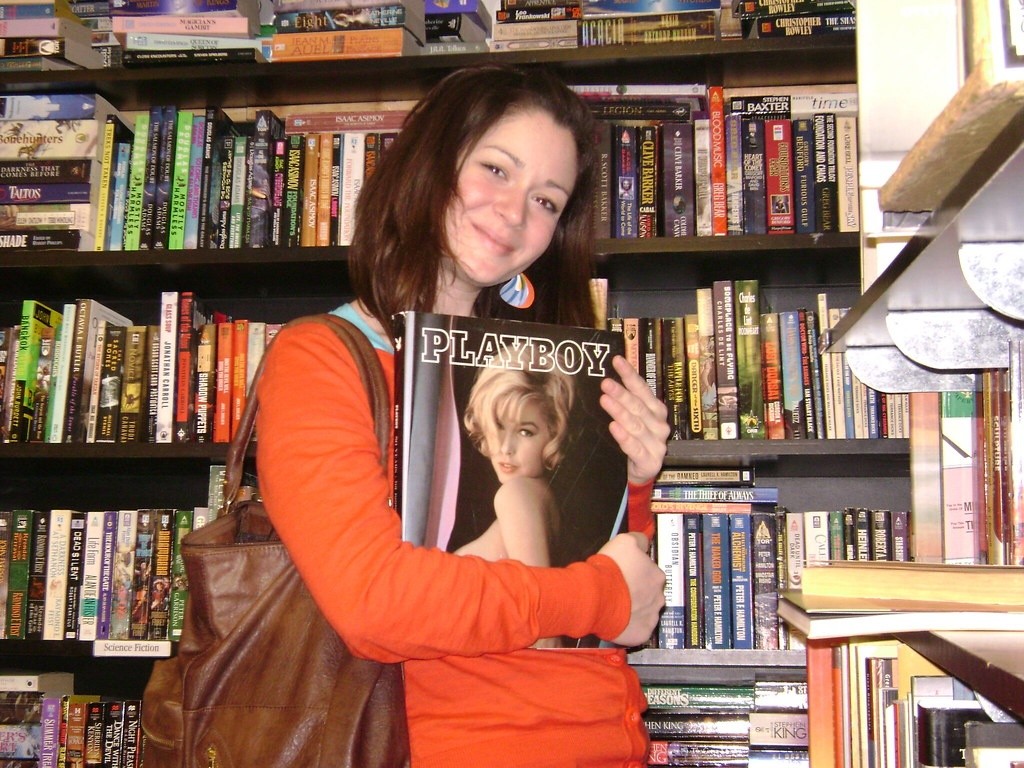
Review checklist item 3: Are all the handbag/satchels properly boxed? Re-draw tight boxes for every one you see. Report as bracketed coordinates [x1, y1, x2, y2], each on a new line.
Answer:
[142, 313, 410, 768]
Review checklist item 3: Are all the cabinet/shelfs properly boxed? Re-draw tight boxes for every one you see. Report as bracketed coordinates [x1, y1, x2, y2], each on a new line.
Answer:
[820, 61, 1024, 719]
[0, 33, 910, 768]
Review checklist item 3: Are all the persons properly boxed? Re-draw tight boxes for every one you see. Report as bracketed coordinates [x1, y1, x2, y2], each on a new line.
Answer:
[255, 64, 672, 768]
[132, 559, 151, 613]
[452, 342, 587, 647]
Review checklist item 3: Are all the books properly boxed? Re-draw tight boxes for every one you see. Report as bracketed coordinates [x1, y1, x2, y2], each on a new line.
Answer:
[2, 0, 1024, 766]
[387, 309, 628, 649]
